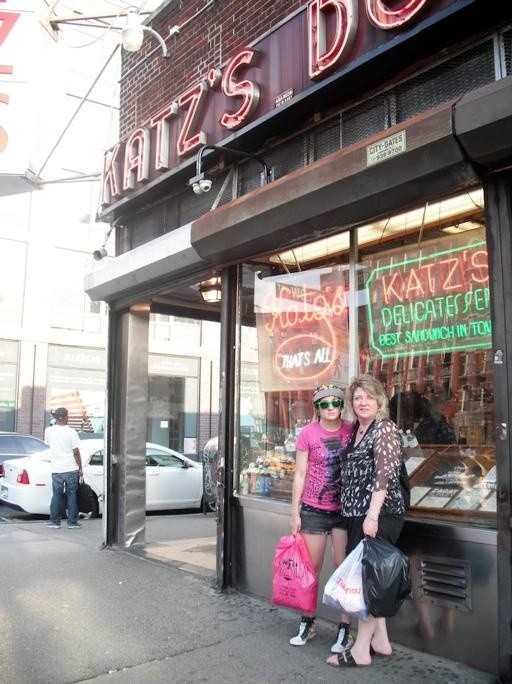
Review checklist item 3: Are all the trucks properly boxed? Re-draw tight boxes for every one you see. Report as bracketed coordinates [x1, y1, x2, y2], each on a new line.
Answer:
[240, 414, 260, 457]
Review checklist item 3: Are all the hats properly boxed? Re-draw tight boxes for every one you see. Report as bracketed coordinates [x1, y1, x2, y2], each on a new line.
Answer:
[311, 384, 345, 404]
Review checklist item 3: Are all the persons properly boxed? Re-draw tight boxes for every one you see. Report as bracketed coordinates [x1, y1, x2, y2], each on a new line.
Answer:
[327, 375, 407, 668]
[43, 406, 85, 529]
[285, 385, 359, 652]
[391, 389, 457, 635]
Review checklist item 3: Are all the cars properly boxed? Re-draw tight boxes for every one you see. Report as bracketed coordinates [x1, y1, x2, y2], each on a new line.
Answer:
[0, 430, 49, 468]
[1, 439, 216, 523]
[258, 440, 295, 480]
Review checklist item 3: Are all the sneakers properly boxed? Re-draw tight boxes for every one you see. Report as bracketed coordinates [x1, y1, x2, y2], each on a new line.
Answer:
[46, 522, 61, 529]
[67, 523, 83, 529]
[330, 622, 354, 653]
[289, 615, 317, 646]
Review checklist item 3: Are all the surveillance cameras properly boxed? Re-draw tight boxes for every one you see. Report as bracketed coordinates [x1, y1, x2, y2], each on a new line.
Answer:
[199, 179, 212, 192]
[192, 183, 202, 194]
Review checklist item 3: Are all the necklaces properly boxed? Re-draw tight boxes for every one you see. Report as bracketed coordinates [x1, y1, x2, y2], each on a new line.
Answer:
[358, 425, 368, 433]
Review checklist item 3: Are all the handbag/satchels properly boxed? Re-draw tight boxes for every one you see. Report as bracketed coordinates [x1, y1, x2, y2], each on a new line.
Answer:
[399, 462, 411, 509]
[62, 475, 99, 520]
[270, 534, 319, 613]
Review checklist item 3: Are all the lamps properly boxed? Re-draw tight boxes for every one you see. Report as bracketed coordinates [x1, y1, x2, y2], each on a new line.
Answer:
[94, 211, 125, 261]
[197, 268, 223, 304]
[119, 9, 172, 58]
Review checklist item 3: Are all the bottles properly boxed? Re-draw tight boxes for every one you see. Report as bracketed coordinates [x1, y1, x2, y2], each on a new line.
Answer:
[295, 419, 303, 435]
[260, 469, 272, 497]
[285, 433, 295, 457]
[397, 428, 422, 456]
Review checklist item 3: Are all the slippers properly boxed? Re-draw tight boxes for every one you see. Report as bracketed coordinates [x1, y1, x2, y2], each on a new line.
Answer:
[326, 650, 372, 667]
[370, 644, 392, 656]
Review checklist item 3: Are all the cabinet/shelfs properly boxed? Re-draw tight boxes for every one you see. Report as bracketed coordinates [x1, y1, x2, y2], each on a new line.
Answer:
[361, 333, 494, 444]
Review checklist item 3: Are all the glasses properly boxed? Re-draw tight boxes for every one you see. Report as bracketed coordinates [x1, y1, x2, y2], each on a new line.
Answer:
[316, 399, 343, 409]
